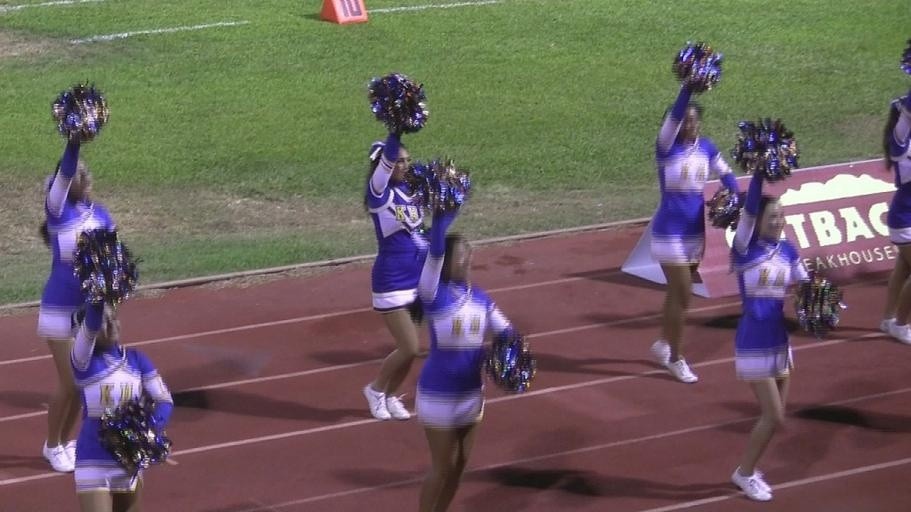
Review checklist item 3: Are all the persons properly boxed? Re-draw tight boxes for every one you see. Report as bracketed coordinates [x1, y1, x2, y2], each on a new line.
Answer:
[880, 95, 911, 344]
[69, 300, 174, 512]
[363, 131, 431, 421]
[35, 137, 117, 472]
[414, 211, 513, 512]
[731, 176, 807, 502]
[648, 84, 736, 383]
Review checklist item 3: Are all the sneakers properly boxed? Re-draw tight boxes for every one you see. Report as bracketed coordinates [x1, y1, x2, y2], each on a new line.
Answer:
[652, 340, 698, 384]
[364, 384, 410, 421]
[880, 318, 910, 344]
[731, 467, 772, 502]
[42, 440, 76, 474]
